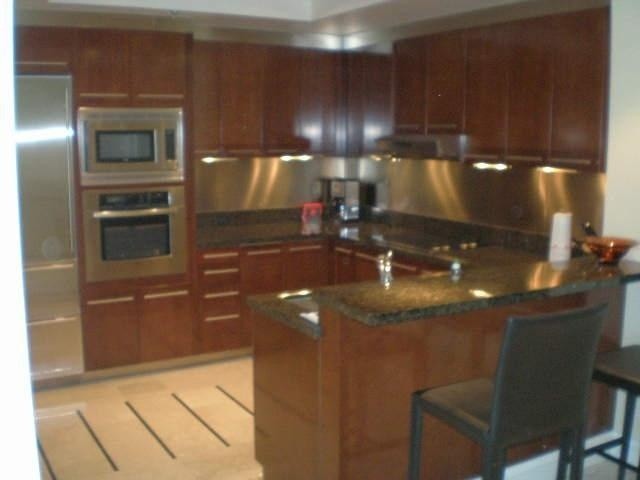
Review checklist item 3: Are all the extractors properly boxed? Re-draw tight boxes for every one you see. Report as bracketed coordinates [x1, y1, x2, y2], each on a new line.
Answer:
[374, 133, 465, 161]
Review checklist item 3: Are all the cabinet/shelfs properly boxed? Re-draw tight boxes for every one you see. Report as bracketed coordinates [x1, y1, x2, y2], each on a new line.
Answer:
[16, 26, 75, 76]
[69, 30, 192, 104]
[333, 245, 421, 291]
[185, 40, 394, 154]
[77, 275, 197, 370]
[196, 240, 331, 365]
[388, 8, 607, 170]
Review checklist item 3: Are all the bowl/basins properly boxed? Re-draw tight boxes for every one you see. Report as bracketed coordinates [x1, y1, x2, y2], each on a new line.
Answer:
[585, 236, 638, 264]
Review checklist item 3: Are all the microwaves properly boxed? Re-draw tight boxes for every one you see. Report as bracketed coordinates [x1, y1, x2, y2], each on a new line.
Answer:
[85, 118, 179, 174]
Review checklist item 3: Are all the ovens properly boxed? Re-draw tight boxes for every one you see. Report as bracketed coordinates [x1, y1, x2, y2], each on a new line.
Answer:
[80, 185, 189, 283]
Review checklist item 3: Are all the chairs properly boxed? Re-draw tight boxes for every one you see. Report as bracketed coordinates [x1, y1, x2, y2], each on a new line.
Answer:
[555, 342, 638, 480]
[410, 299, 610, 480]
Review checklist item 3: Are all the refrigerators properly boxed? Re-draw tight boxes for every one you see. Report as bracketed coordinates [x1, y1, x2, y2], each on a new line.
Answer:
[13, 73, 89, 384]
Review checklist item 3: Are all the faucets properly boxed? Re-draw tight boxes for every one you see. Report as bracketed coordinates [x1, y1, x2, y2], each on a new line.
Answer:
[373, 247, 396, 279]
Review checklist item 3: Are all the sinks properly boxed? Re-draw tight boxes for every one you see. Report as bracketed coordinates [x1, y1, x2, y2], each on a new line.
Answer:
[299, 308, 320, 325]
